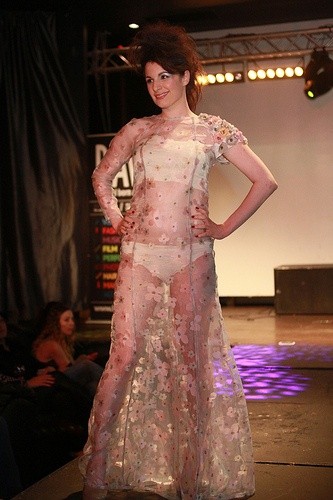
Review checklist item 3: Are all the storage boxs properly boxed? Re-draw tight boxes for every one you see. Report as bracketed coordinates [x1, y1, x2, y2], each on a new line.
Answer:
[274, 263, 333, 314]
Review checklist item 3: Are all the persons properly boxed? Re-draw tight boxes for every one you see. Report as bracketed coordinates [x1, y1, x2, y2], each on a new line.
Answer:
[24, 303, 104, 400]
[78, 22, 280, 499]
[0, 313, 89, 500]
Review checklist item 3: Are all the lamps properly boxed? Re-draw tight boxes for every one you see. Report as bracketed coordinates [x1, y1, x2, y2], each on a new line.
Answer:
[246, 56, 305, 81]
[303, 47, 333, 101]
[195, 62, 245, 87]
